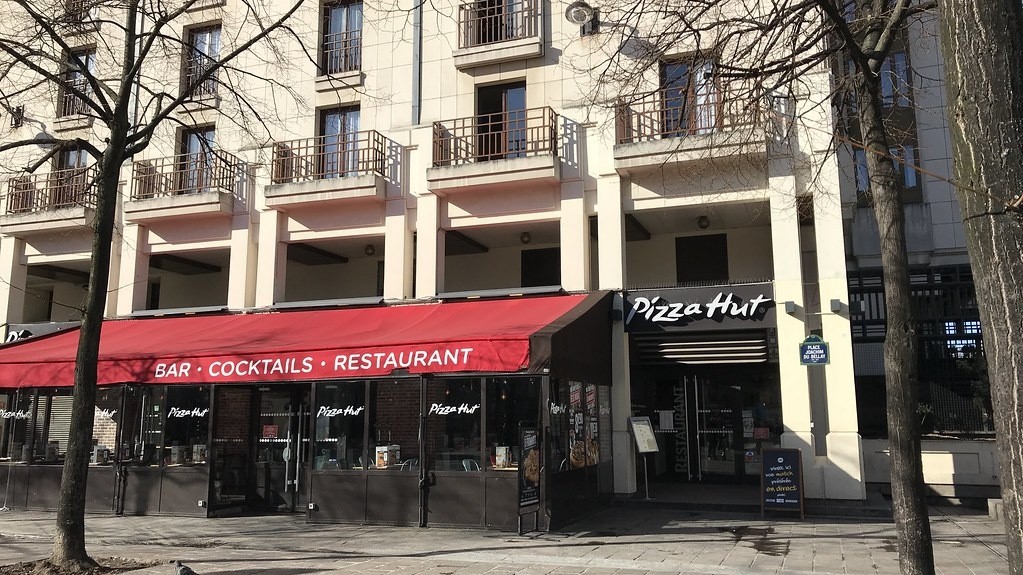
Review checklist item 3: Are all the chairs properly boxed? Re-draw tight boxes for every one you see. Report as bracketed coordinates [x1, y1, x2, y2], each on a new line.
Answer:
[461, 459, 481, 472]
[334, 452, 421, 471]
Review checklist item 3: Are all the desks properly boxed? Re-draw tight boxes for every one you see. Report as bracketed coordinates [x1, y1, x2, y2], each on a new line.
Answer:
[485, 460, 519, 471]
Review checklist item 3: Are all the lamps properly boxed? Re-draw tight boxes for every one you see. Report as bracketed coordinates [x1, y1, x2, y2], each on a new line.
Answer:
[565, 1, 601, 38]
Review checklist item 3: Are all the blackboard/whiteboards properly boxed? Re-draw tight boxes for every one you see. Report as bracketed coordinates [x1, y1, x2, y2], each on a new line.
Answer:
[759, 447, 805, 521]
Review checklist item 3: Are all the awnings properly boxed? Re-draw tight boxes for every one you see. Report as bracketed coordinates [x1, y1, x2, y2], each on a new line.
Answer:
[0, 290, 615, 389]
